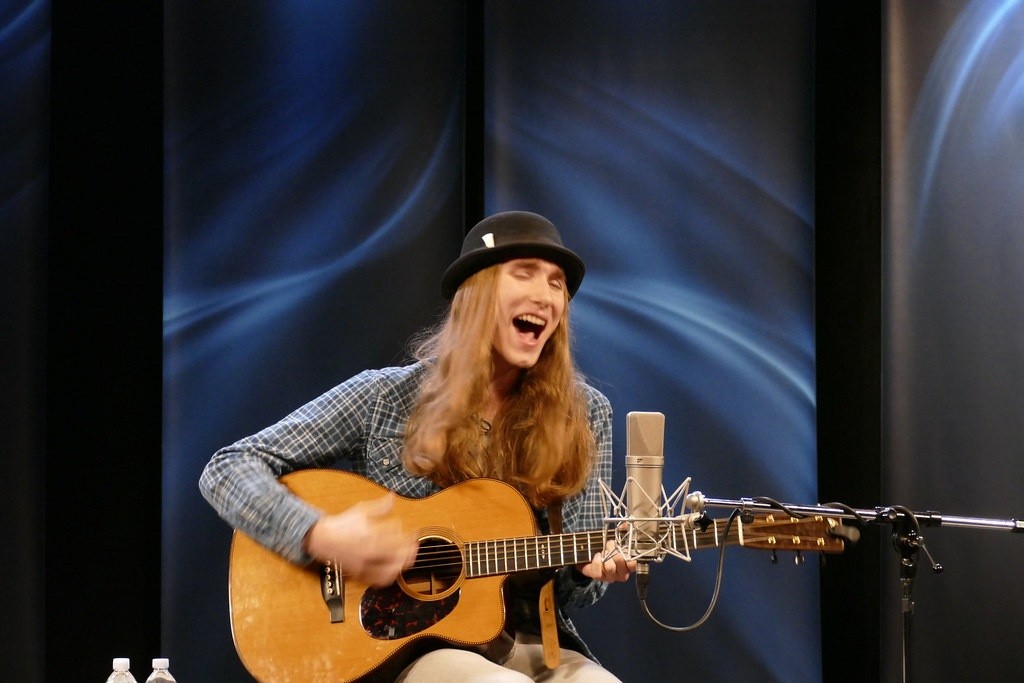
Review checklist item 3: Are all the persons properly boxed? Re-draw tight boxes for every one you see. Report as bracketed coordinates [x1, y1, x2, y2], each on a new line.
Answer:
[194, 209, 636, 682]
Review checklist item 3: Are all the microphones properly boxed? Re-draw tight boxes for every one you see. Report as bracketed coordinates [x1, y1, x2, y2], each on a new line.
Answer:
[625, 409, 665, 599]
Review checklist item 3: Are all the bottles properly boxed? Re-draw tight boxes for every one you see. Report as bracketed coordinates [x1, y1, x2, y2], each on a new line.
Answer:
[105, 657, 137, 683]
[145, 658, 177, 683]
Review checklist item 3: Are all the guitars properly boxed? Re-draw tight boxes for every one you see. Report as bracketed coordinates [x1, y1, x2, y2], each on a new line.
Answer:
[224, 466, 844, 683]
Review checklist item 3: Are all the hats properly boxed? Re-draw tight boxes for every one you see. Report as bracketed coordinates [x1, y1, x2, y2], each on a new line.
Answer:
[438, 211, 587, 302]
